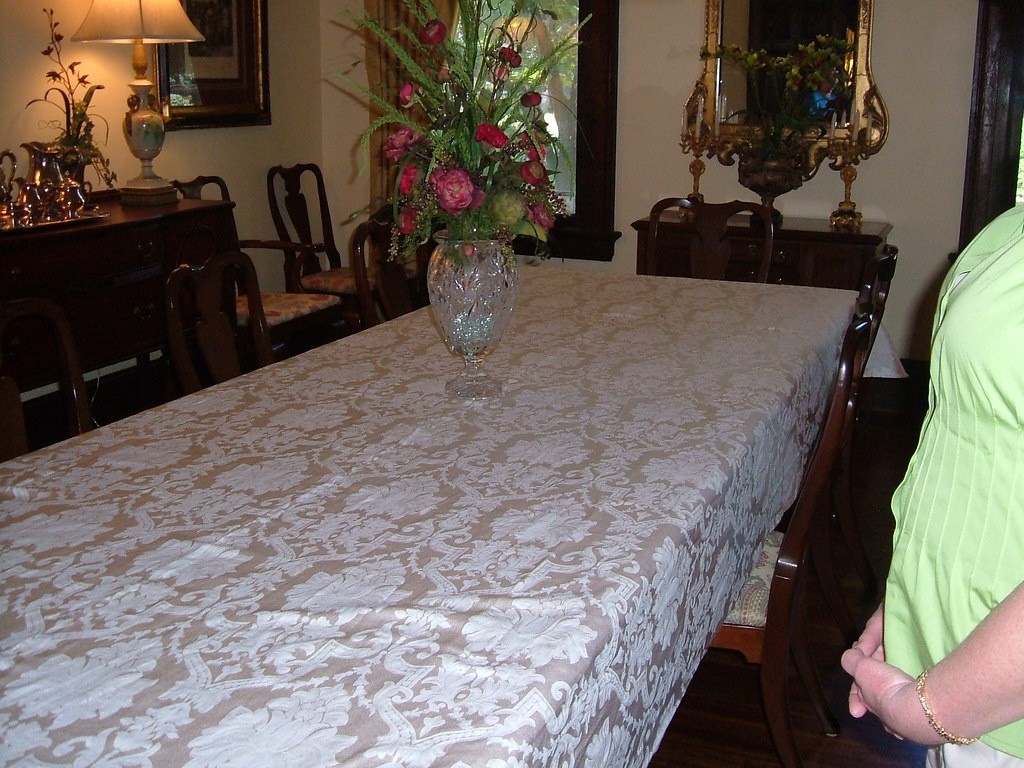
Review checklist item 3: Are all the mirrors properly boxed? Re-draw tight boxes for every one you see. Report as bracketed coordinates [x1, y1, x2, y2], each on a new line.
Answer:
[678, 0, 891, 182]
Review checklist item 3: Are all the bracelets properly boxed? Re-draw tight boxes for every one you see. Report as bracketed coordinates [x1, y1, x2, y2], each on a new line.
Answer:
[916, 670, 980, 746]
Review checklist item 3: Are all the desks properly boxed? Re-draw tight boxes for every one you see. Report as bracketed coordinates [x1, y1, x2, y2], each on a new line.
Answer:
[0, 258, 909, 768]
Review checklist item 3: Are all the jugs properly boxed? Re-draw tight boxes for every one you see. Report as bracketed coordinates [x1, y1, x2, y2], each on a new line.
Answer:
[53, 171, 85, 221]
[20, 140, 62, 223]
[12, 177, 54, 224]
[0, 147, 18, 220]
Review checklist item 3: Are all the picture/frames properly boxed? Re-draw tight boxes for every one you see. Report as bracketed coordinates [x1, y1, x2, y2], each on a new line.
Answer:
[152, 0, 272, 132]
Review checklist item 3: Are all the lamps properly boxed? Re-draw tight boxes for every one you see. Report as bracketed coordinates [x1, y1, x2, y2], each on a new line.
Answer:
[71, 0, 205, 208]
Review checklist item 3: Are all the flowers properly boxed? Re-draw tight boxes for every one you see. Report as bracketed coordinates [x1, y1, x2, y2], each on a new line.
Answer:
[23, 6, 119, 188]
[331, 0, 595, 272]
[693, 34, 859, 159]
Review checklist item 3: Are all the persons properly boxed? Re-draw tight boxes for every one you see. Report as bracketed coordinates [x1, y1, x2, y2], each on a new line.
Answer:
[841, 203, 1024, 768]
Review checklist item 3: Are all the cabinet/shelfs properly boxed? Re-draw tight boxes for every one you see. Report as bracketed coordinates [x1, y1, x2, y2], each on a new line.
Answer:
[630, 209, 893, 294]
[0, 190, 245, 395]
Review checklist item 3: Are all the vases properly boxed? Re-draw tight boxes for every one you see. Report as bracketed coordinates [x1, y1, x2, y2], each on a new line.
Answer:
[0, 142, 111, 234]
[426, 227, 517, 401]
[738, 156, 804, 230]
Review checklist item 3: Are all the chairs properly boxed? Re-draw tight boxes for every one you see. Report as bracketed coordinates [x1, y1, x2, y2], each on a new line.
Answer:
[267, 163, 419, 337]
[167, 174, 347, 368]
[349, 202, 434, 329]
[644, 197, 774, 282]
[706, 311, 873, 768]
[0, 298, 94, 464]
[806, 240, 899, 648]
